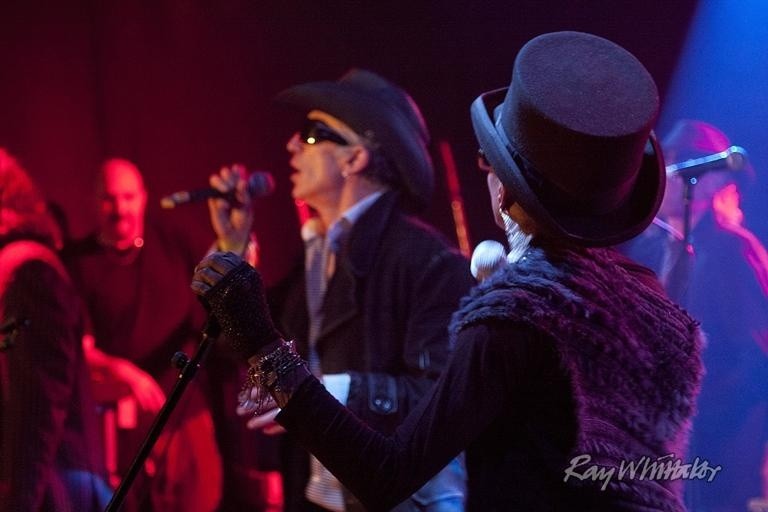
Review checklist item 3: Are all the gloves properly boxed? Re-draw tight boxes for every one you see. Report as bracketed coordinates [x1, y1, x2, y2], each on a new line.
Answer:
[188, 249, 283, 362]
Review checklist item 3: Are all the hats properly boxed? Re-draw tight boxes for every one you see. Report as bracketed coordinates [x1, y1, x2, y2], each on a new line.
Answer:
[467, 29, 670, 250]
[283, 65, 437, 199]
[661, 117, 732, 158]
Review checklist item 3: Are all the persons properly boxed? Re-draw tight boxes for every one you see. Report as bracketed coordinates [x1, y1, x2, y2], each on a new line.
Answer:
[0, 31, 768, 512]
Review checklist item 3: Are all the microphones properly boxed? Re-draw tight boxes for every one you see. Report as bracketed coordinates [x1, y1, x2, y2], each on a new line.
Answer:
[665, 146, 748, 183]
[466, 240, 507, 295]
[160, 172, 277, 209]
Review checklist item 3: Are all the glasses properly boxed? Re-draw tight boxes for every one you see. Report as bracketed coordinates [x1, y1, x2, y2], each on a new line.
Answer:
[297, 112, 352, 147]
